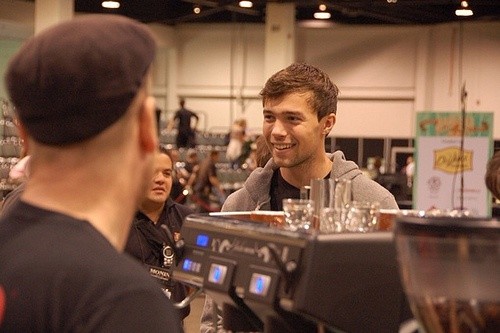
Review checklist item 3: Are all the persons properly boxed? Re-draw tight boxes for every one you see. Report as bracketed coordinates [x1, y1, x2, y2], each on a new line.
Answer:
[0, 13, 184, 333]
[200, 63, 401, 333]
[484, 149, 499, 200]
[123, 146, 194, 318]
[0, 87, 415, 212]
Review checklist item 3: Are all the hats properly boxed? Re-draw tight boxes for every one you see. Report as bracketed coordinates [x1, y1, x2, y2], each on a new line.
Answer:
[3, 13, 157, 149]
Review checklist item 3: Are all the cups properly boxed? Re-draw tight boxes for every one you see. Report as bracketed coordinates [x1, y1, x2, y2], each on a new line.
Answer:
[320, 207, 347, 234]
[283, 199, 314, 230]
[300, 178, 351, 229]
[347, 200, 380, 232]
